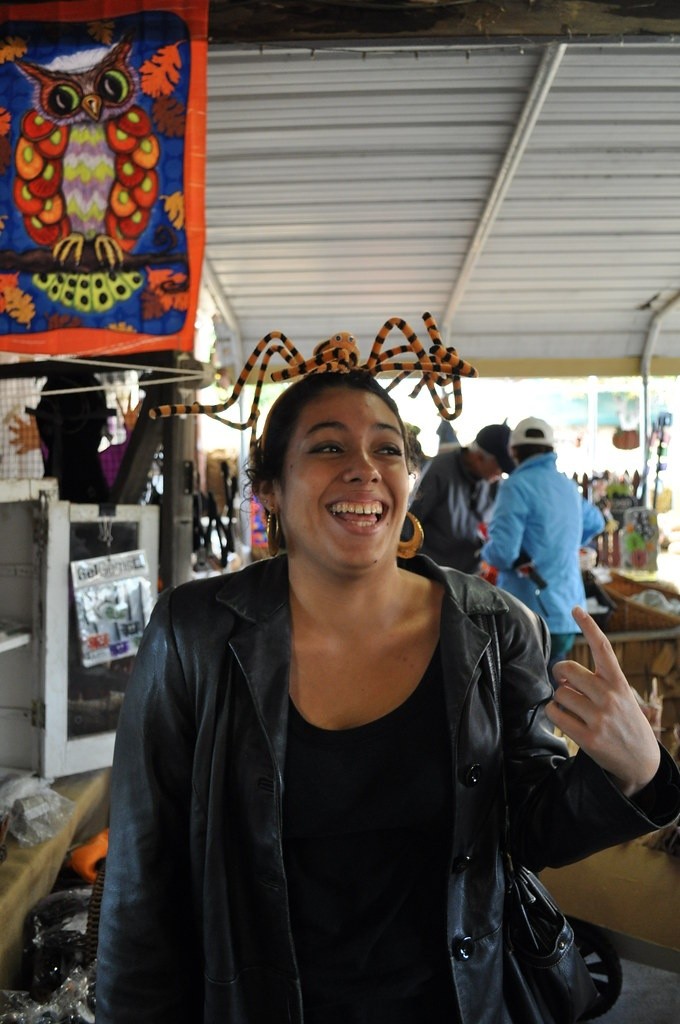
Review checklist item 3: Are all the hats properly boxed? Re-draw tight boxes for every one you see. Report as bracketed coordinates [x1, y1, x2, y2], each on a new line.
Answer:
[476, 424, 517, 474]
[507, 416, 555, 448]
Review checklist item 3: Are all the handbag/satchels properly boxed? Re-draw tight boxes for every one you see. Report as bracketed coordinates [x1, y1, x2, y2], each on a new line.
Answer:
[500, 863, 600, 1024]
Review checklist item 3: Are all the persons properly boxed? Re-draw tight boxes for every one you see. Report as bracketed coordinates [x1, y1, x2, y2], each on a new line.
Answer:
[402, 424, 518, 577]
[91, 367, 680, 1023]
[483, 416, 604, 686]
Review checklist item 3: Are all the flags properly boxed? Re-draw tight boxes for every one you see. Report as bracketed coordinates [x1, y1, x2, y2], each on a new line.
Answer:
[2, 2, 206, 355]
